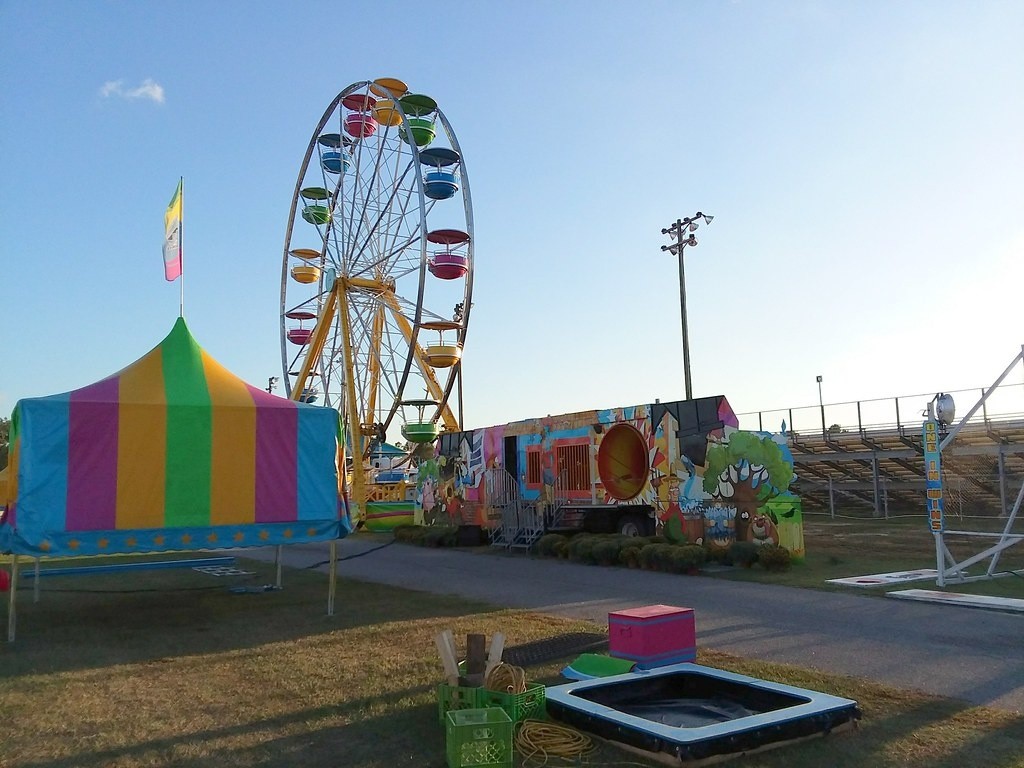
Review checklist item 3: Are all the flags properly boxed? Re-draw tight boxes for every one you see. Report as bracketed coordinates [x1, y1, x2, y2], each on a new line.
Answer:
[162, 179, 183, 281]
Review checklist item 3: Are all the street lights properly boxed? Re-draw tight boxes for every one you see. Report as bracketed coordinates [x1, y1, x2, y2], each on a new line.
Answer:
[660, 211, 715, 400]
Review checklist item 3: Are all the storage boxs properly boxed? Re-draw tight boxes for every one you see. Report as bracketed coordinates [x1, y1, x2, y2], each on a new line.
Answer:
[609, 603, 697, 670]
[438, 658, 547, 768]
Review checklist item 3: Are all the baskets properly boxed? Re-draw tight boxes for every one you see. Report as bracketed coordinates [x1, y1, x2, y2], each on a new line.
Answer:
[445, 707, 513, 768]
[475, 682, 545, 724]
[438, 676, 477, 724]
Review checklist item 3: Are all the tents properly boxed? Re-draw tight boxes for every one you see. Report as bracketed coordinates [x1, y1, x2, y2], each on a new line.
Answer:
[0, 317, 350, 641]
